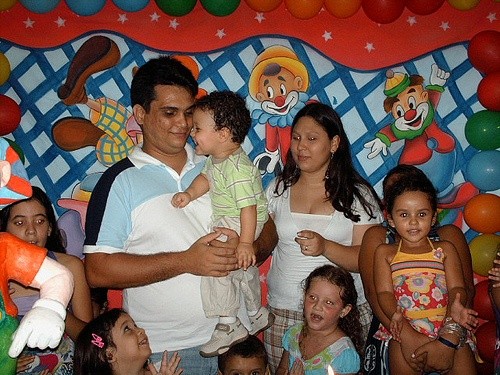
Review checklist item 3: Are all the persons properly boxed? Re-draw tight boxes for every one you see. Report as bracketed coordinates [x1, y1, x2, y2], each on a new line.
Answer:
[359, 164, 478, 375]
[263, 102, 384, 375]
[82, 56, 276, 375]
[274, 264, 365, 375]
[0, 137, 74, 375]
[218, 334, 271, 375]
[0, 183, 94, 375]
[73, 308, 183, 375]
[170, 90, 275, 358]
[488, 252, 500, 375]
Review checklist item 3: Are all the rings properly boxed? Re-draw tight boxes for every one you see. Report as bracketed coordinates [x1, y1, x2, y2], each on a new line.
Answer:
[305, 246, 308, 250]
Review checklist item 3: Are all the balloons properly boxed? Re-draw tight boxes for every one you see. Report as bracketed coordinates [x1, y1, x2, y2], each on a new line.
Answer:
[0, 0, 478, 24]
[466, 149, 500, 191]
[477, 73, 500, 110]
[467, 30, 500, 75]
[465, 110, 500, 150]
[0, 52, 25, 165]
[463, 194, 500, 234]
[468, 234, 500, 361]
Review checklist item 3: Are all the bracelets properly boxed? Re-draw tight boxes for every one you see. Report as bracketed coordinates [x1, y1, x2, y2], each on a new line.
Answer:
[438, 336, 458, 349]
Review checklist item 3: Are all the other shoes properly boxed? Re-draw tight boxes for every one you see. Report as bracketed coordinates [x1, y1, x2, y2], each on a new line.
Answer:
[199, 317, 249, 358]
[249, 306, 275, 338]
